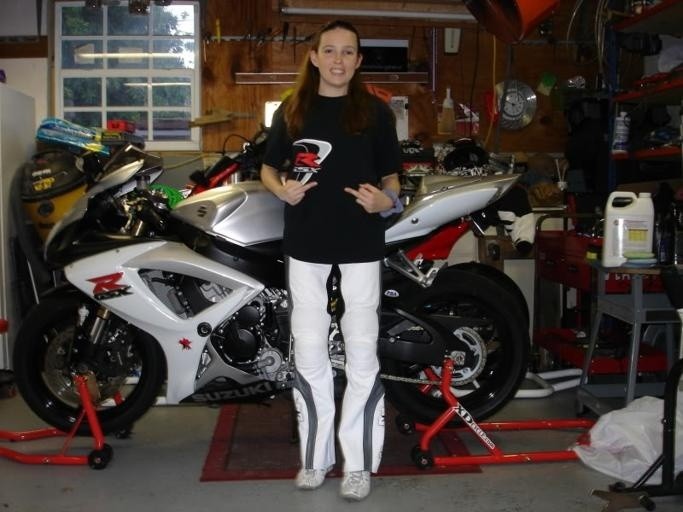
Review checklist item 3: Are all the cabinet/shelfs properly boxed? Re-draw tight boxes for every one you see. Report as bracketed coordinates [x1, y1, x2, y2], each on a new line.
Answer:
[530, 212, 664, 375]
[570, 259, 677, 420]
[605, 1, 682, 165]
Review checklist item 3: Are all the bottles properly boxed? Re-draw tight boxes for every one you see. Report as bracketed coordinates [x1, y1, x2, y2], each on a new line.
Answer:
[654, 208, 683, 266]
[440, 88, 456, 133]
[610, 112, 631, 154]
[106, 119, 135, 133]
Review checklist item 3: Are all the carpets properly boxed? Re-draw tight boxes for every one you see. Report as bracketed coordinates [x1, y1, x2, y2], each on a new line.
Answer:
[196, 396, 484, 483]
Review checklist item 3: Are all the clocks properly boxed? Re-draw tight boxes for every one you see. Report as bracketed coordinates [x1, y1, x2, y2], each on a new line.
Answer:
[485, 78, 537, 131]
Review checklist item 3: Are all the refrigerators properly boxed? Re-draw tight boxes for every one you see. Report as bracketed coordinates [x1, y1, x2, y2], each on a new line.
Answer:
[0, 80, 37, 377]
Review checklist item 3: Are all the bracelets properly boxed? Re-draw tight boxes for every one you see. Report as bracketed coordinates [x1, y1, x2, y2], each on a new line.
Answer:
[378, 185, 402, 218]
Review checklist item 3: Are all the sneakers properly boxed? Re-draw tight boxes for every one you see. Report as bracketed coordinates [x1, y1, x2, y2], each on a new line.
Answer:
[292, 461, 336, 490]
[338, 469, 373, 502]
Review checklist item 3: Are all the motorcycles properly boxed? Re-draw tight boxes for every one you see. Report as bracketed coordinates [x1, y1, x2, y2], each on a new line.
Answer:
[12, 139, 530, 433]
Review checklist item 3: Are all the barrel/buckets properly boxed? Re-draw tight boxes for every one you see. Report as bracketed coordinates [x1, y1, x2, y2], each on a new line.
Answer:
[601, 190, 654, 269]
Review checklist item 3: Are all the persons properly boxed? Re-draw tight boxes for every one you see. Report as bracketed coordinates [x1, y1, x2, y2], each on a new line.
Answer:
[257, 21, 402, 501]
